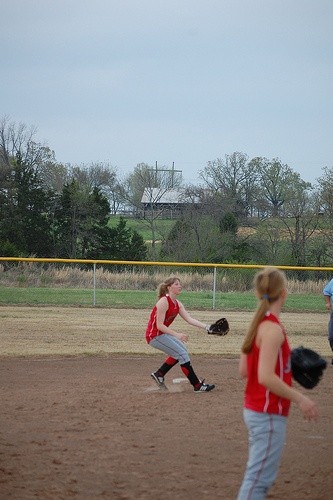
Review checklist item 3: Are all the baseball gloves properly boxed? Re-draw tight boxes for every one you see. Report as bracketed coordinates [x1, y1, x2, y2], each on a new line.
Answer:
[291, 346, 327, 390]
[208, 317, 230, 336]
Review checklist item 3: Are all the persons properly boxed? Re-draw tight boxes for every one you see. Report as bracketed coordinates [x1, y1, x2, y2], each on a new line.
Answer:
[146, 278, 215, 393]
[238, 269, 319, 500]
[323, 279, 333, 364]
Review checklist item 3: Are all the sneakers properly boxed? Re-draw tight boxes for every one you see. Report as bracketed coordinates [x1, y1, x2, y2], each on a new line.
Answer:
[194, 377, 216, 393]
[151, 368, 169, 391]
